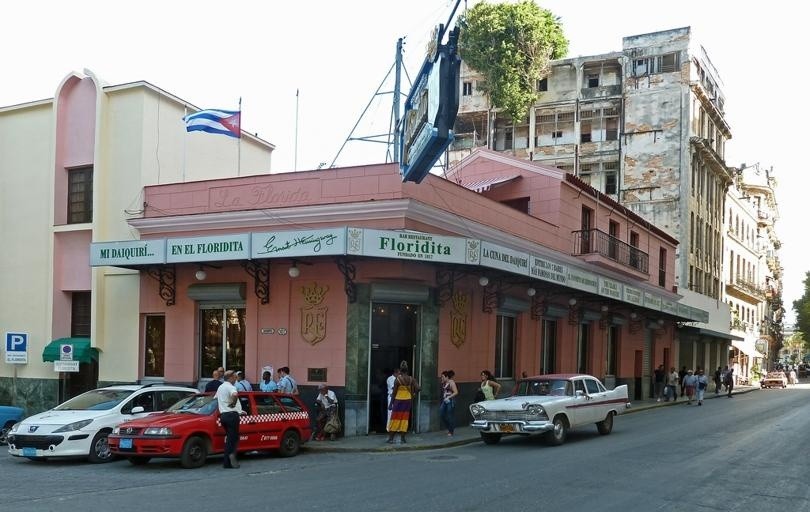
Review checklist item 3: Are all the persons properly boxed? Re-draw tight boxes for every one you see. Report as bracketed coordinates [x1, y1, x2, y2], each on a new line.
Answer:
[313, 384, 339, 441]
[385, 360, 420, 443]
[439, 369, 459, 436]
[205, 366, 300, 407]
[147, 346, 156, 374]
[385, 366, 400, 433]
[540, 384, 550, 394]
[213, 370, 243, 469]
[653, 364, 733, 406]
[788, 368, 797, 385]
[512, 371, 534, 396]
[477, 371, 501, 400]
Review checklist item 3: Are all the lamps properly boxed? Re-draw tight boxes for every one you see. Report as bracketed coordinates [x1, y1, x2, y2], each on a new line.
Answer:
[288, 259, 314, 277]
[196, 264, 222, 280]
[478, 268, 685, 339]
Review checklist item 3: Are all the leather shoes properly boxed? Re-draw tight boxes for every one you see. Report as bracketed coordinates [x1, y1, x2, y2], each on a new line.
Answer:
[230, 454, 240, 468]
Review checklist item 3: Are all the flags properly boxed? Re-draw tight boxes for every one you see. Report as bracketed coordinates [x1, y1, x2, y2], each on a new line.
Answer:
[183, 108, 240, 138]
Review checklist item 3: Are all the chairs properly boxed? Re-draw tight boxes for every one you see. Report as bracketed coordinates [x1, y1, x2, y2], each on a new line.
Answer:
[135, 394, 152, 411]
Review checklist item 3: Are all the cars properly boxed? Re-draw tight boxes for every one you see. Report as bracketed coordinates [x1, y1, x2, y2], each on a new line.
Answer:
[0, 403, 27, 447]
[105, 389, 315, 472]
[758, 363, 810, 390]
[6, 379, 203, 465]
[467, 371, 634, 448]
